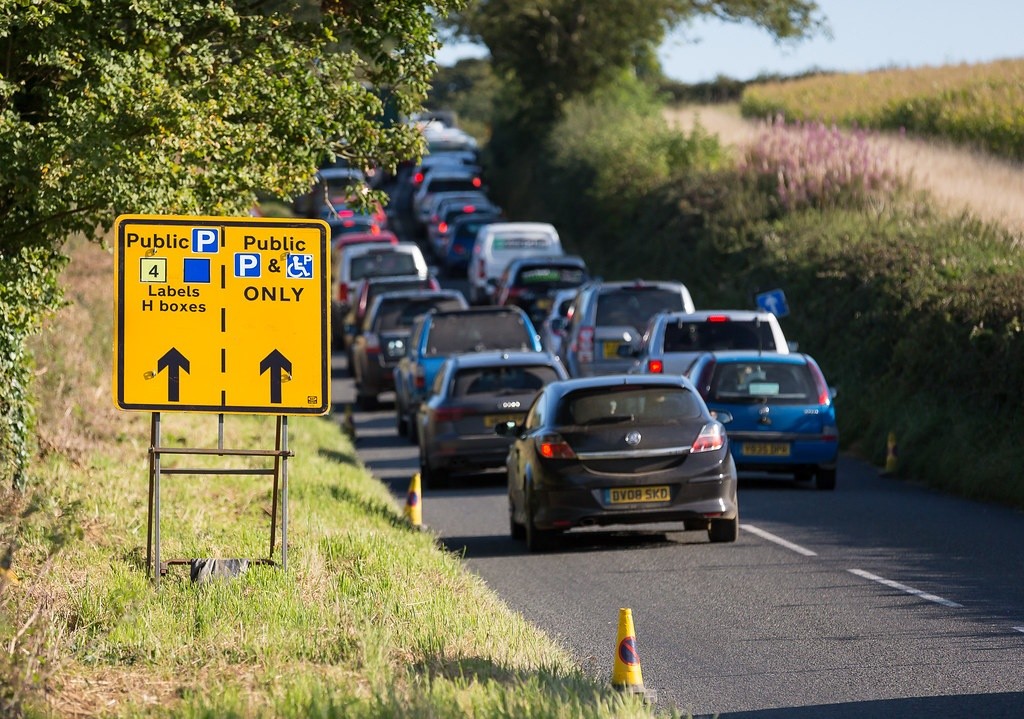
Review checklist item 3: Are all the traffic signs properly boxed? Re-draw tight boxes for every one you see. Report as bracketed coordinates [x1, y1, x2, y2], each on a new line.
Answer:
[113, 214, 331, 416]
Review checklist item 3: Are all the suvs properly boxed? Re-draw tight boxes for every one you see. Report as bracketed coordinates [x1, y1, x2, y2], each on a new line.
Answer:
[342, 290, 485, 411]
[616, 308, 800, 394]
[485, 256, 591, 335]
[494, 374, 739, 554]
[466, 221, 567, 308]
[387, 301, 541, 447]
[549, 277, 695, 383]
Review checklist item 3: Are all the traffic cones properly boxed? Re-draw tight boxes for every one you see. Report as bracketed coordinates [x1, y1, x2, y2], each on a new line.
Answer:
[879, 433, 899, 479]
[605, 606, 658, 707]
[402, 474, 427, 533]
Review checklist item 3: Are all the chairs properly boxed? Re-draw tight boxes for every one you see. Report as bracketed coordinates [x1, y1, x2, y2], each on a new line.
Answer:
[766, 367, 799, 393]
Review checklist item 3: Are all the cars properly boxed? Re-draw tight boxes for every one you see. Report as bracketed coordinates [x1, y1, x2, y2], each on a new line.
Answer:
[531, 287, 580, 369]
[411, 346, 571, 491]
[683, 348, 837, 489]
[314, 110, 504, 377]
